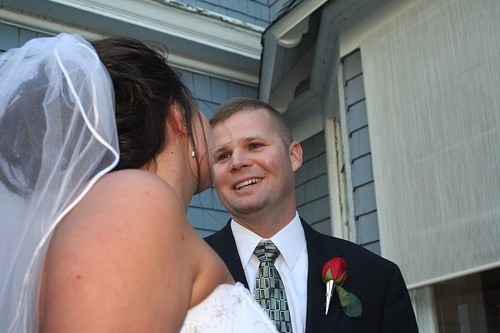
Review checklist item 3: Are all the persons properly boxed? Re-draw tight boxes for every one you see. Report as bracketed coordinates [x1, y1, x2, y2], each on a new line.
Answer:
[0, 31, 278, 333]
[203, 96, 419, 333]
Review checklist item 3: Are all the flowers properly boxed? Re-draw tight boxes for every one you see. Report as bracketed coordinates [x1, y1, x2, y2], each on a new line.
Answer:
[322, 257, 347, 285]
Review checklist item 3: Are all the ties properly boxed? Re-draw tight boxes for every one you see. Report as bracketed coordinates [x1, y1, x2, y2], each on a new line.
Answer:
[252, 239, 292, 333]
